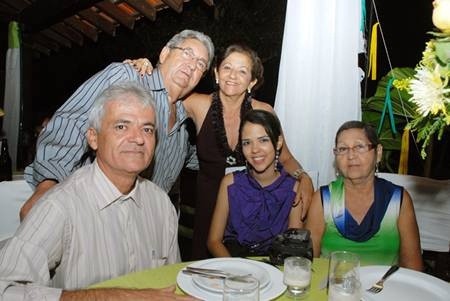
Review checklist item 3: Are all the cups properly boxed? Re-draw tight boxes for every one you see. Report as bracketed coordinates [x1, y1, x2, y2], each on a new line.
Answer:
[328, 250, 362, 301]
[283, 256, 312, 299]
[222, 275, 259, 301]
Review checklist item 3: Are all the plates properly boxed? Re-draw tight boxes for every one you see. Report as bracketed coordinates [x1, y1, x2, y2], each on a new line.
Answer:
[360, 266, 450, 301]
[192, 260, 272, 293]
[176, 257, 287, 301]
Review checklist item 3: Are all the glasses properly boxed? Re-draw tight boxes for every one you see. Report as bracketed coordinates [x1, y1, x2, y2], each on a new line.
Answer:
[331, 140, 380, 157]
[166, 44, 211, 76]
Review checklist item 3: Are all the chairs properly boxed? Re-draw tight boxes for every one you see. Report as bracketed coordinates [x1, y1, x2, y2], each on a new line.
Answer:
[0, 179, 34, 242]
[373, 173, 450, 252]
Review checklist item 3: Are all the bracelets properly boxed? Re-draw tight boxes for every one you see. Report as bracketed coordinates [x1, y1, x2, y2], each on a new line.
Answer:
[293, 169, 307, 179]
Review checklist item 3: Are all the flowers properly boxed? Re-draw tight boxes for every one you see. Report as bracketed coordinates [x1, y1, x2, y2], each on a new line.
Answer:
[407, 0, 450, 160]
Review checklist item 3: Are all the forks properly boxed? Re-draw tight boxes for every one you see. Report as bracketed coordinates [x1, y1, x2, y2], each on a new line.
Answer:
[366, 263, 400, 293]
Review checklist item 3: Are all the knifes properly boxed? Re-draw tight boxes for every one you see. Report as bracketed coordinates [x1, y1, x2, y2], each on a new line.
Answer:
[182, 270, 249, 283]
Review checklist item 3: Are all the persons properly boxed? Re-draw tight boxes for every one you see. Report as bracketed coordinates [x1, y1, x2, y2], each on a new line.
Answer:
[0, 81, 204, 301]
[305, 121, 423, 272]
[124, 46, 314, 260]
[18, 29, 215, 225]
[208, 109, 303, 258]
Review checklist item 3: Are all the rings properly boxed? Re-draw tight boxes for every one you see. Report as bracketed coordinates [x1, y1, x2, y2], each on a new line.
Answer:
[144, 62, 148, 67]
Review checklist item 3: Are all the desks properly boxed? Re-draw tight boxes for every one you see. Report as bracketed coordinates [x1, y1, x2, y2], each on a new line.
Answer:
[82, 256, 349, 301]
